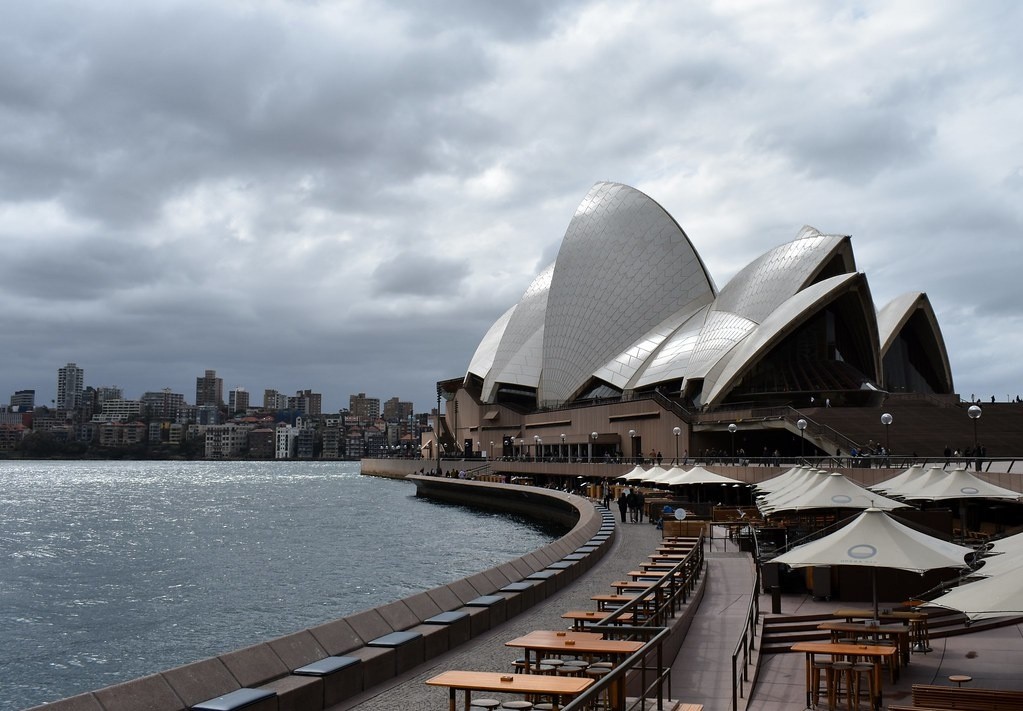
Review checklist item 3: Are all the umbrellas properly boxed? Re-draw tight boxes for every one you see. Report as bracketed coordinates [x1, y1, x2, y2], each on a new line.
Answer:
[751, 465, 1023, 645]
[617, 465, 746, 504]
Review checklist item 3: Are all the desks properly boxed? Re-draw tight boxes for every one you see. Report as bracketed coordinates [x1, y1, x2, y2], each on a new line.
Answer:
[505, 630, 646, 711]
[590, 595, 655, 619]
[639, 537, 702, 603]
[817, 622, 911, 669]
[560, 610, 634, 640]
[834, 609, 928, 650]
[627, 571, 682, 618]
[611, 580, 670, 624]
[791, 642, 897, 711]
[426, 670, 595, 711]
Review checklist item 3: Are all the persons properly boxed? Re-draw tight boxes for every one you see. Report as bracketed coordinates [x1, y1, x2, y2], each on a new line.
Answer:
[638, 449, 663, 465]
[698, 447, 782, 469]
[835, 440, 890, 468]
[517, 451, 588, 463]
[826, 397, 831, 408]
[618, 488, 645, 524]
[811, 396, 815, 408]
[944, 443, 986, 468]
[603, 487, 611, 510]
[682, 449, 687, 465]
[655, 385, 668, 398]
[414, 467, 472, 480]
[595, 395, 602, 405]
[603, 451, 623, 464]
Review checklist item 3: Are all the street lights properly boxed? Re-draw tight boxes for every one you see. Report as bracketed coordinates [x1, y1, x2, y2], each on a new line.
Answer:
[968, 405, 982, 471]
[592, 432, 598, 464]
[385, 446, 389, 458]
[629, 429, 636, 465]
[477, 441, 480, 457]
[538, 439, 541, 460]
[391, 446, 394, 459]
[797, 419, 807, 467]
[444, 443, 447, 458]
[403, 445, 407, 459]
[534, 435, 539, 462]
[510, 437, 515, 461]
[520, 440, 523, 461]
[1007, 394, 1009, 402]
[504, 441, 509, 460]
[881, 414, 893, 468]
[436, 382, 443, 473]
[410, 409, 413, 453]
[673, 427, 682, 465]
[728, 424, 737, 466]
[560, 434, 566, 464]
[465, 442, 469, 455]
[490, 441, 494, 460]
[454, 397, 459, 457]
[380, 446, 383, 459]
[411, 445, 414, 460]
[396, 445, 400, 459]
[418, 445, 422, 459]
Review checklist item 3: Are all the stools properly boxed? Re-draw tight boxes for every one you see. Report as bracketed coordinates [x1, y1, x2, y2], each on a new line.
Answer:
[470, 699, 564, 711]
[511, 658, 613, 711]
[949, 675, 972, 687]
[813, 619, 926, 711]
[903, 601, 923, 612]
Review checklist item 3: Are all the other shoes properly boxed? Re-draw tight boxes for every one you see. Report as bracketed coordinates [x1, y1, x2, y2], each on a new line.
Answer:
[630, 521, 633, 523]
[633, 518, 637, 523]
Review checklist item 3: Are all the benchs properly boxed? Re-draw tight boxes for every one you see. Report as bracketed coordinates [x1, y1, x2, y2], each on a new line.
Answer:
[888, 683, 1023, 711]
